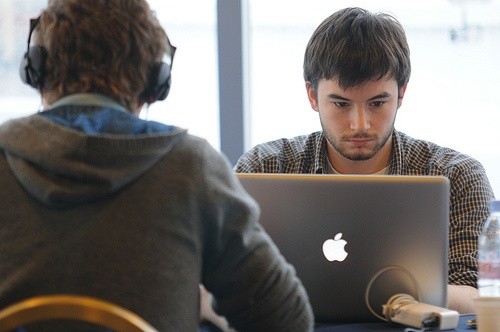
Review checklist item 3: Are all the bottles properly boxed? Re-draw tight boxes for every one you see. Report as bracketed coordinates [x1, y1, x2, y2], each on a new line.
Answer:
[476, 200, 500, 297]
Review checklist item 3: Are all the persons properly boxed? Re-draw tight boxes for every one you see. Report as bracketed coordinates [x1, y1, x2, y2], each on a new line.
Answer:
[235, 7, 495, 314]
[0, 0, 314, 332]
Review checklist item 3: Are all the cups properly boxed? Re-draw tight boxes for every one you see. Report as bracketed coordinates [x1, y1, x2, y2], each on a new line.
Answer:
[472, 298, 500, 332]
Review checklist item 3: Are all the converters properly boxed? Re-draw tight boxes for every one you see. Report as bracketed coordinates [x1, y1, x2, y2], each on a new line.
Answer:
[381, 301, 460, 331]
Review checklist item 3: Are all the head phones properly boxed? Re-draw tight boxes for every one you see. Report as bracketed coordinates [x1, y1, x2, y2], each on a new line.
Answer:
[18, 14, 176, 105]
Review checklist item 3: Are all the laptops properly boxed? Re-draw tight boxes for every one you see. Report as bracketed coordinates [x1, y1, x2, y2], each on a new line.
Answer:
[234, 172, 451, 323]
